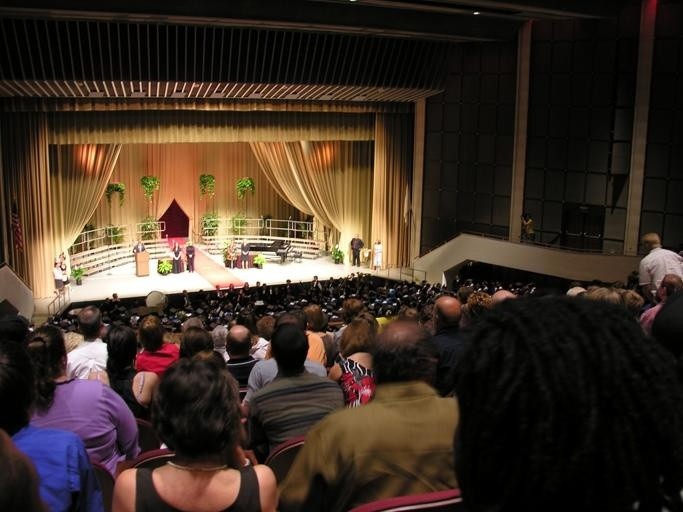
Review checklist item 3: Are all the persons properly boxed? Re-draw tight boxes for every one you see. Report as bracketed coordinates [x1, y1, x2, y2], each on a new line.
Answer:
[654, 295, 683, 354]
[226, 238, 237, 267]
[522, 214, 535, 240]
[277, 323, 460, 511]
[186, 241, 195, 272]
[0, 430, 51, 512]
[53, 260, 64, 296]
[224, 324, 258, 387]
[133, 240, 145, 252]
[372, 240, 383, 272]
[47, 273, 341, 341]
[172, 242, 182, 274]
[133, 316, 177, 378]
[66, 306, 110, 387]
[112, 355, 279, 512]
[23, 326, 142, 475]
[240, 239, 250, 269]
[639, 233, 683, 295]
[88, 328, 159, 420]
[1, 351, 106, 512]
[341, 272, 647, 327]
[329, 325, 377, 409]
[454, 296, 683, 512]
[350, 233, 364, 266]
[61, 256, 70, 286]
[178, 327, 225, 371]
[641, 274, 683, 334]
[248, 325, 344, 454]
[2, 314, 30, 352]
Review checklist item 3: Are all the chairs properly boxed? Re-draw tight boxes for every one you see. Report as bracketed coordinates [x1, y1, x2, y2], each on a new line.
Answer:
[124, 450, 179, 471]
[348, 489, 462, 512]
[89, 459, 114, 512]
[266, 437, 307, 482]
[136, 417, 160, 447]
[226, 360, 255, 381]
[237, 389, 251, 399]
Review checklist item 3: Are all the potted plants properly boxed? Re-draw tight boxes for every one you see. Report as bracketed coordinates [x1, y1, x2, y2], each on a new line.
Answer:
[141, 174, 160, 202]
[234, 175, 257, 200]
[71, 266, 85, 285]
[105, 179, 125, 209]
[330, 247, 342, 264]
[140, 216, 161, 246]
[157, 259, 172, 275]
[222, 242, 238, 268]
[82, 224, 99, 250]
[198, 172, 215, 201]
[104, 225, 125, 248]
[254, 254, 264, 269]
[259, 211, 271, 239]
[201, 211, 219, 245]
[230, 211, 248, 240]
